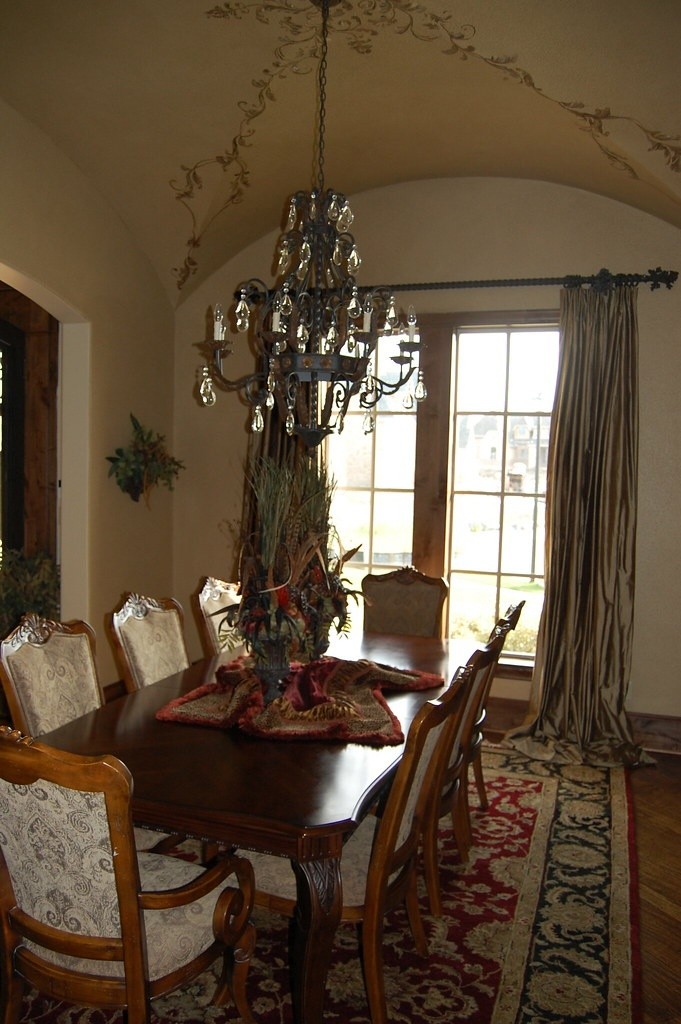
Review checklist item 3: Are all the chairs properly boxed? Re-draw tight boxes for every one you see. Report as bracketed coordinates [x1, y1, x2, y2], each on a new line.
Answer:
[366, 636, 503, 917]
[1, 611, 184, 853]
[463, 599, 527, 829]
[213, 674, 471, 1022]
[104, 588, 190, 693]
[0, 727, 256, 1024]
[190, 574, 247, 657]
[360, 562, 448, 638]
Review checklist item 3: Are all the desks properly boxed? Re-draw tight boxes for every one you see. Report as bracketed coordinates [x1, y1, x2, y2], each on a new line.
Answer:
[44, 634, 479, 1024]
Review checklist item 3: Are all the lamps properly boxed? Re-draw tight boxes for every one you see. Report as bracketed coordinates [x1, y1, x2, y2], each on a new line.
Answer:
[196, 0, 428, 433]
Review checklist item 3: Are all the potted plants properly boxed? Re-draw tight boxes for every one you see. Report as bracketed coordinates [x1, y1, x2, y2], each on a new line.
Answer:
[108, 416, 185, 509]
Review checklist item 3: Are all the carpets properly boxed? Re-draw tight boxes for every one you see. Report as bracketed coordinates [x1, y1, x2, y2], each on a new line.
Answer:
[13, 744, 642, 1024]
